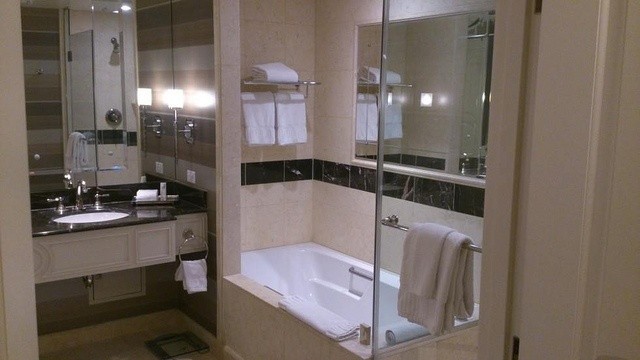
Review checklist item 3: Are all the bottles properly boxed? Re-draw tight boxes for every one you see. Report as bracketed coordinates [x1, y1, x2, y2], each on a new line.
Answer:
[160, 182, 166, 201]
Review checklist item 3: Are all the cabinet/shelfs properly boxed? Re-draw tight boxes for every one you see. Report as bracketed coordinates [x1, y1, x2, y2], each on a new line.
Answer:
[83, 267, 147, 308]
[174, 212, 209, 262]
[33, 218, 176, 286]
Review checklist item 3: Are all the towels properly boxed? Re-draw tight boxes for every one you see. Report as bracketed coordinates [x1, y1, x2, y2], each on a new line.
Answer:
[174, 259, 208, 296]
[397, 221, 474, 336]
[65, 133, 89, 173]
[275, 91, 307, 147]
[356, 92, 378, 142]
[251, 63, 298, 82]
[241, 91, 276, 147]
[359, 65, 400, 84]
[385, 95, 404, 140]
[135, 188, 158, 201]
[279, 295, 360, 342]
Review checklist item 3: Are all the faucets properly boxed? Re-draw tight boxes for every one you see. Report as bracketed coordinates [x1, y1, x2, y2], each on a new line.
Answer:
[63, 167, 73, 189]
[75, 180, 87, 211]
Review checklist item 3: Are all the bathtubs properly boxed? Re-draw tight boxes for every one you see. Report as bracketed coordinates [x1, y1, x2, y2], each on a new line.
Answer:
[240, 241, 420, 328]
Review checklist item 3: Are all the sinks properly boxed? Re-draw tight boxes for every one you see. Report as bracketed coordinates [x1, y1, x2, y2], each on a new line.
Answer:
[53, 211, 132, 224]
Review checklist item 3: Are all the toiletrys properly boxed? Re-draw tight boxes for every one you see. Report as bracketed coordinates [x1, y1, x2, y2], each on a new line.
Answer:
[160, 180, 168, 200]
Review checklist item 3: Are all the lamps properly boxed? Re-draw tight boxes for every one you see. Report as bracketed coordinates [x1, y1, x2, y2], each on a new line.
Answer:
[137, 88, 162, 138]
[167, 88, 194, 144]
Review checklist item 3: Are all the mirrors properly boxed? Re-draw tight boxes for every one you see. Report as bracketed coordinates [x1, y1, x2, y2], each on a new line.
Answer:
[20, 1, 177, 193]
[351, 7, 495, 189]
